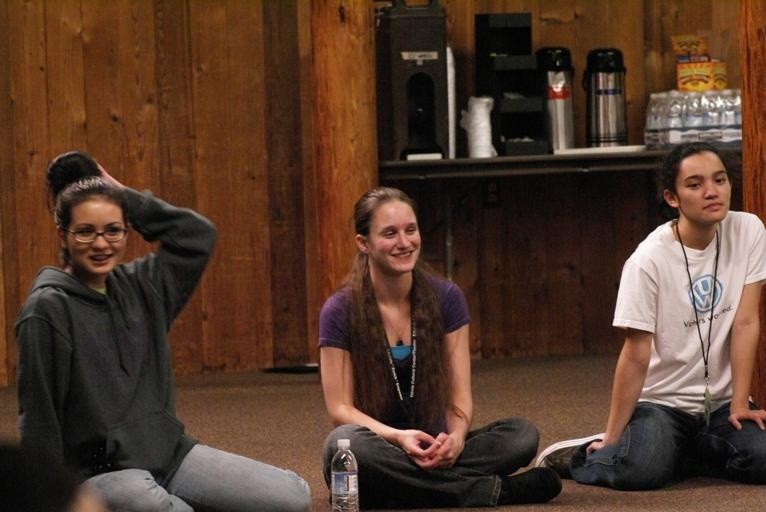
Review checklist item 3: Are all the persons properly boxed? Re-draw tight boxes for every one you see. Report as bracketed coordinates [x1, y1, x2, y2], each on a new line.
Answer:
[12, 153, 311, 512]
[318, 188, 562, 507]
[536, 140, 765, 489]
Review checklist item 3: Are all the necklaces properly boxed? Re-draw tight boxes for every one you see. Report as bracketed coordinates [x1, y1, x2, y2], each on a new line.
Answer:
[374, 290, 411, 346]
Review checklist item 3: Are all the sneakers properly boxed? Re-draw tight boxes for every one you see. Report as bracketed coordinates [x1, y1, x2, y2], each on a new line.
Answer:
[534, 434, 608, 477]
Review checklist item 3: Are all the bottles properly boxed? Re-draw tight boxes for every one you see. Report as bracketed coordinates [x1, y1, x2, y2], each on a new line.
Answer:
[582, 47, 630, 148]
[538, 45, 575, 149]
[331, 438, 360, 512]
[468, 97, 492, 158]
[643, 87, 742, 150]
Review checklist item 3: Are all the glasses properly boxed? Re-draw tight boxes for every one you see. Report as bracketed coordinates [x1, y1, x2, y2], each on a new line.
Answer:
[60, 225, 128, 243]
